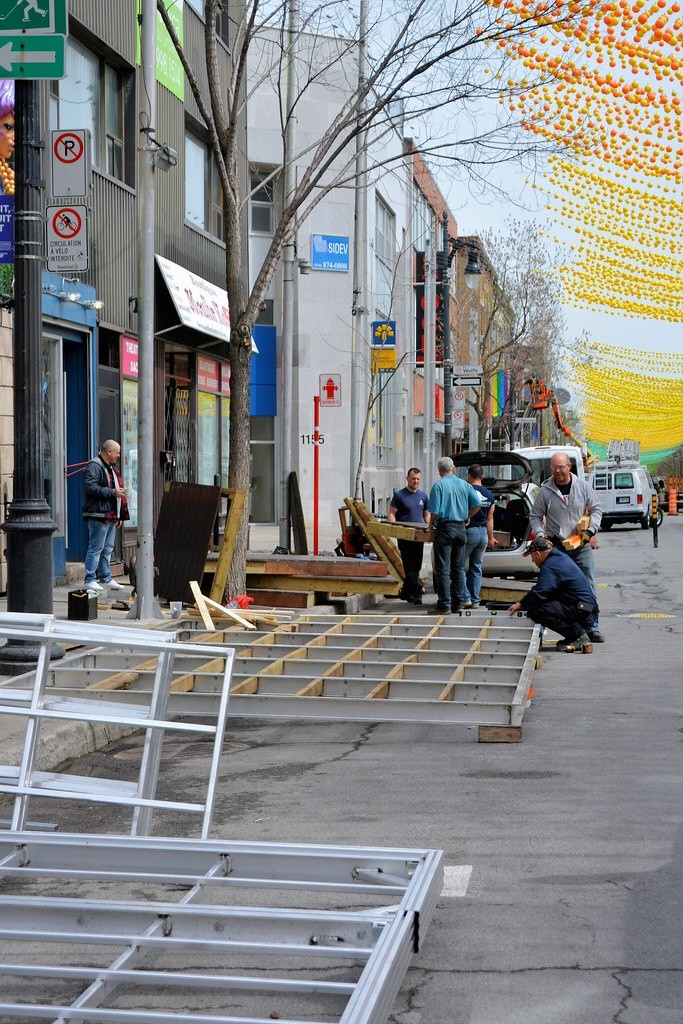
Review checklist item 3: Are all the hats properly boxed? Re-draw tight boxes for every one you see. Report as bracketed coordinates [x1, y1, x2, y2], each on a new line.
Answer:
[523, 536, 553, 557]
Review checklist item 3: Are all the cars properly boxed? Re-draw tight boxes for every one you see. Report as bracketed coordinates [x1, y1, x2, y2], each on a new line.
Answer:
[444, 449, 547, 580]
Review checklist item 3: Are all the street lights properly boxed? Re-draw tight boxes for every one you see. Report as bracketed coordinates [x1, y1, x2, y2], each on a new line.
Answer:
[436, 210, 484, 462]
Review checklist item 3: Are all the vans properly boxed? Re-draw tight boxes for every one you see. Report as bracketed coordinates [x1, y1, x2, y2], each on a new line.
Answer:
[511, 445, 586, 492]
[587, 459, 665, 533]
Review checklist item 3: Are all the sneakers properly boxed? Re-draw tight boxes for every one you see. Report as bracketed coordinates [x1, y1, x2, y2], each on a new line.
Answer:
[99, 579, 124, 589]
[83, 582, 103, 591]
[588, 632, 605, 642]
[556, 632, 588, 651]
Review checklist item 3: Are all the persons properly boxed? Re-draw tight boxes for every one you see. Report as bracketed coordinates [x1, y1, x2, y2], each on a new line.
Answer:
[461, 464, 498, 608]
[82, 440, 129, 590]
[387, 468, 430, 605]
[529, 452, 605, 642]
[426, 457, 481, 615]
[507, 538, 599, 651]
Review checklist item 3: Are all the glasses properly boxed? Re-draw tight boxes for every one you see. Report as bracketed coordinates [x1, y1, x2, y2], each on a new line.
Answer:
[550, 463, 569, 471]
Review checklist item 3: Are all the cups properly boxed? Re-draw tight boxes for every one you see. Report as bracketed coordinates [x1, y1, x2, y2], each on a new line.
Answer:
[170, 602, 182, 619]
[123, 489, 131, 498]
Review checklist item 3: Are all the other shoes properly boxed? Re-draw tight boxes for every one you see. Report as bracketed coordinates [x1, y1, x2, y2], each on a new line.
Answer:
[463, 600, 472, 607]
[451, 606, 463, 613]
[413, 593, 423, 604]
[427, 607, 451, 615]
[472, 601, 480, 609]
[397, 590, 413, 603]
